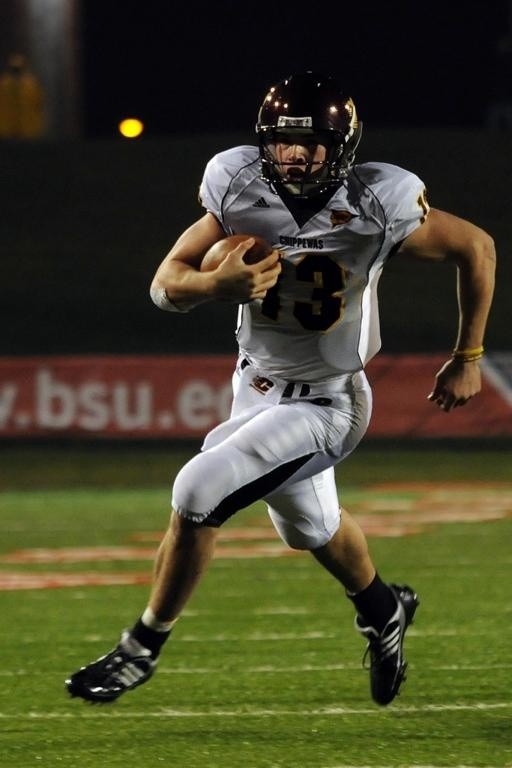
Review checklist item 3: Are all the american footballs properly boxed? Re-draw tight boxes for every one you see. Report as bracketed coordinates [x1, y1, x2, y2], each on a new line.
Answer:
[200, 235, 271, 307]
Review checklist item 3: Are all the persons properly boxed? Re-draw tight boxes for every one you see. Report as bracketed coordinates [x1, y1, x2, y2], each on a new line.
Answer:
[66, 67, 498, 704]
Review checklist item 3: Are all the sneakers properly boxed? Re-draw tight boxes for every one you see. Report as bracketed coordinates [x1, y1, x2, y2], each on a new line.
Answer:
[64, 644, 161, 702]
[354, 583, 419, 705]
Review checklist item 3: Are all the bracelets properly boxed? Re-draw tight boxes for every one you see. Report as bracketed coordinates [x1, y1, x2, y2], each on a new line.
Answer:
[451, 345, 487, 364]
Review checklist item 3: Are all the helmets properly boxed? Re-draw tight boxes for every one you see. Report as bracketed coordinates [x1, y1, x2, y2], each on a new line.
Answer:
[254, 71, 359, 199]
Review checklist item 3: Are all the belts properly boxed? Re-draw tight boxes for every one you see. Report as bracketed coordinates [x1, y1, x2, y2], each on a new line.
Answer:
[240, 359, 352, 397]
